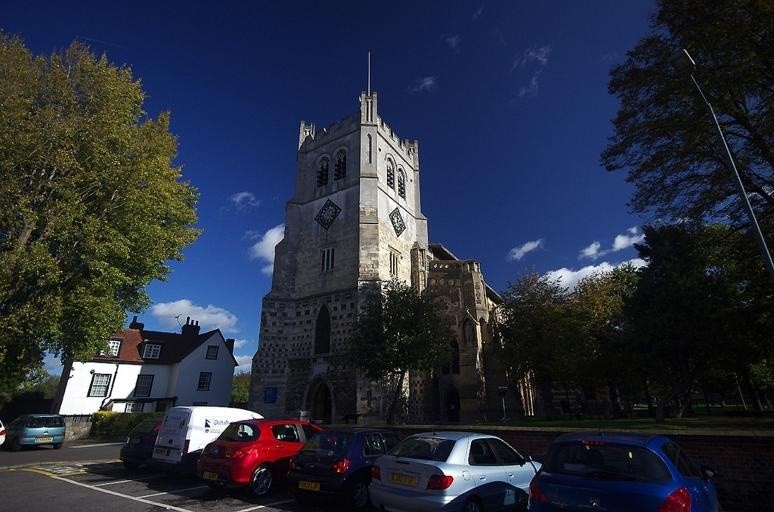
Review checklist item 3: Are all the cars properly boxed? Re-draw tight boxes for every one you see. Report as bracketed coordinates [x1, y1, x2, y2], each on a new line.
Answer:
[288, 428, 412, 512]
[197, 417, 337, 500]
[527, 431, 722, 512]
[119, 418, 167, 473]
[0, 413, 66, 452]
[367, 430, 543, 512]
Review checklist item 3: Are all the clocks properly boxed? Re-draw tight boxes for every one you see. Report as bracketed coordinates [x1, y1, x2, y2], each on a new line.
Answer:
[315, 199, 339, 229]
[387, 207, 406, 236]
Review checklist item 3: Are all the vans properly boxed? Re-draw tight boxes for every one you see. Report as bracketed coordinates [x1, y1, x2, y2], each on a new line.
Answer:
[151, 405, 265, 480]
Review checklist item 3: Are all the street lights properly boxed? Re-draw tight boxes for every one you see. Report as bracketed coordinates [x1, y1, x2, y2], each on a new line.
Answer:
[672, 48, 774, 278]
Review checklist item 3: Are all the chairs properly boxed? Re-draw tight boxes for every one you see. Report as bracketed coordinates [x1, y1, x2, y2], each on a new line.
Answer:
[472, 443, 489, 464]
[284, 429, 297, 442]
